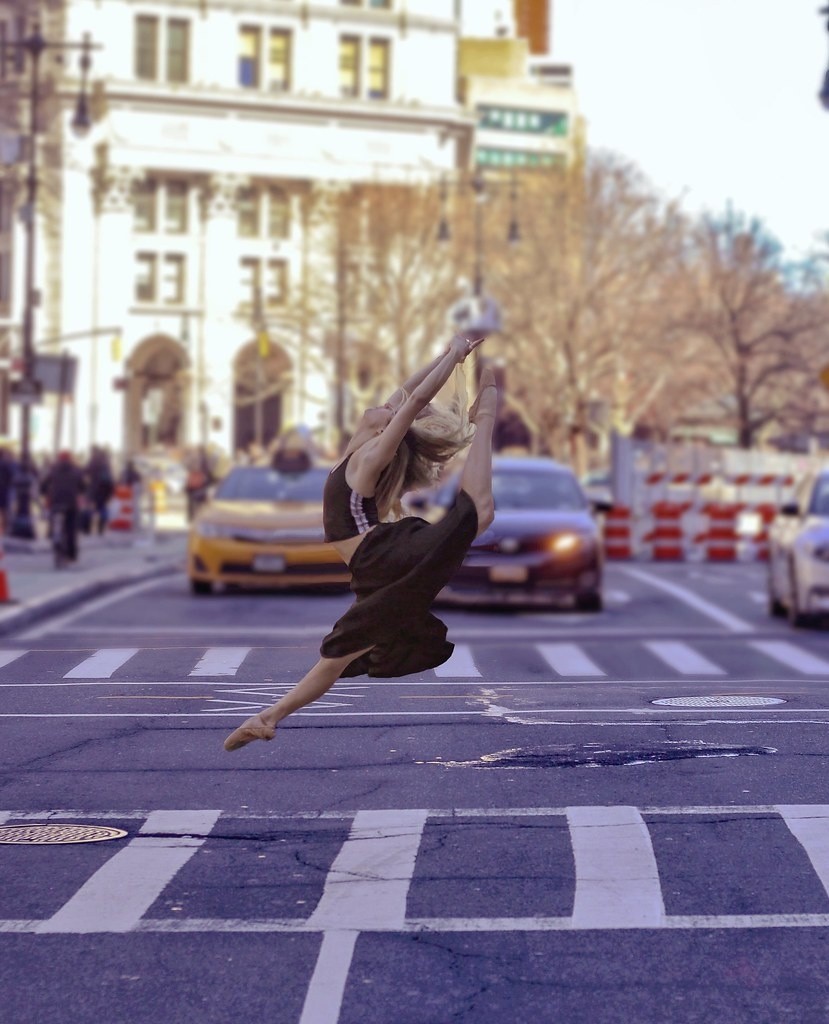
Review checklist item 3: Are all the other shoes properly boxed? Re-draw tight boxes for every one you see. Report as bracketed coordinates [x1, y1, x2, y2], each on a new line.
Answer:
[468, 365, 497, 424]
[222, 714, 278, 753]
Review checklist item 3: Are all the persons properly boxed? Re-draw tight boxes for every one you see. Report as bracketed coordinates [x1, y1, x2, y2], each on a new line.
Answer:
[182, 447, 214, 522]
[40, 451, 81, 562]
[0, 446, 42, 540]
[221, 337, 498, 751]
[83, 444, 111, 537]
[122, 459, 141, 484]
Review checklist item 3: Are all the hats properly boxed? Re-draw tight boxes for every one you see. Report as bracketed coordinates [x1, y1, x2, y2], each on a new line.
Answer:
[58, 450, 71, 461]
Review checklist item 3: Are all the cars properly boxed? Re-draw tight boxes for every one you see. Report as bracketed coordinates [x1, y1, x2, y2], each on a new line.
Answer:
[189, 469, 370, 585]
[766, 470, 828, 619]
[417, 458, 605, 614]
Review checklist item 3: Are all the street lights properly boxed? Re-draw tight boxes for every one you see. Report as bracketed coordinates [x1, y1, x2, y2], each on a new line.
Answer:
[0, 22, 105, 541]
